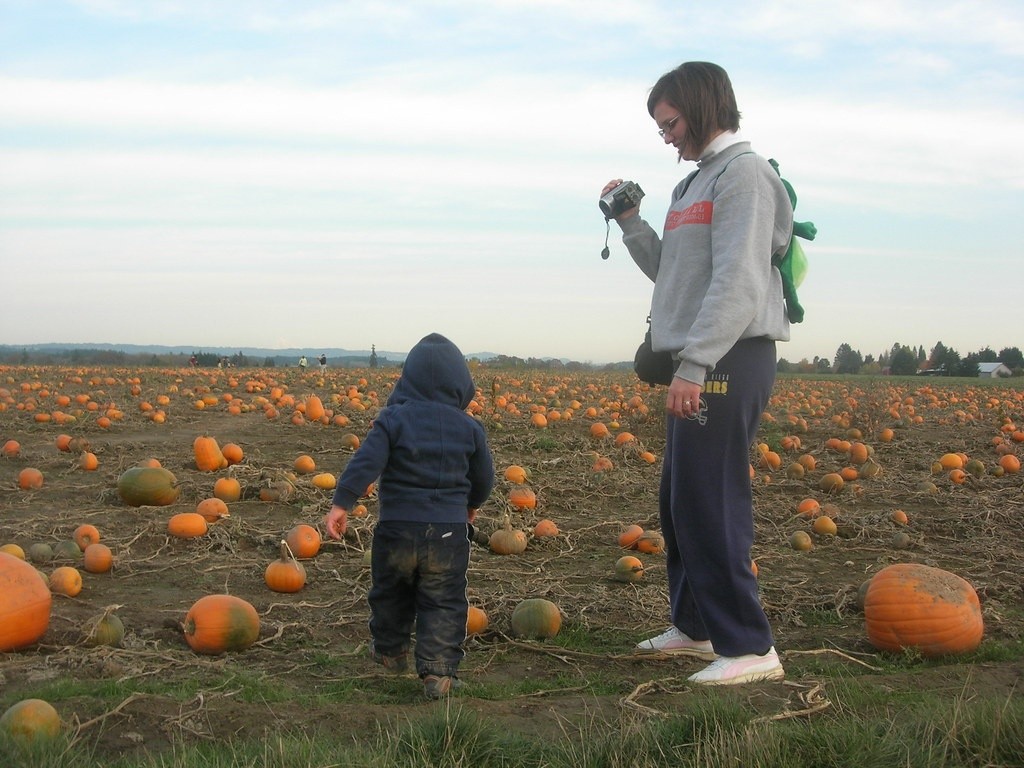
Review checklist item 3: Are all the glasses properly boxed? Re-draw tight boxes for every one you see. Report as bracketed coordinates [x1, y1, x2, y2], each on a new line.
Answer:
[658, 114, 682, 137]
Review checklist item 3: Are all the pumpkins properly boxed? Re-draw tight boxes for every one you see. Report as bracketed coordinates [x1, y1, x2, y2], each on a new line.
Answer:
[0, 368, 1024, 750]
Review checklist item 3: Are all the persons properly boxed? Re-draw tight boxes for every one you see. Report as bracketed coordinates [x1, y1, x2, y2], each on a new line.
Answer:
[322, 332, 493, 702]
[598, 60, 796, 686]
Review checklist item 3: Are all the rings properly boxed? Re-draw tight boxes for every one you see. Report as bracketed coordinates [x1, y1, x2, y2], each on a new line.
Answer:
[682, 399, 693, 406]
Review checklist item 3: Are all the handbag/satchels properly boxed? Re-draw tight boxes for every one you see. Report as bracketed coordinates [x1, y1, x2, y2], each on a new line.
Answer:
[633, 332, 674, 387]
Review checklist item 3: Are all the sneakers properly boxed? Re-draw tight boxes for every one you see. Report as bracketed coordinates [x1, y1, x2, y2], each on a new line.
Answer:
[423, 675, 450, 698]
[368, 639, 407, 672]
[687, 645, 784, 685]
[637, 626, 719, 661]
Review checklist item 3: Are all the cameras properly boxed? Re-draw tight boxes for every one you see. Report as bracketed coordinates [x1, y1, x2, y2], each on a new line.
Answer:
[598, 181, 646, 219]
